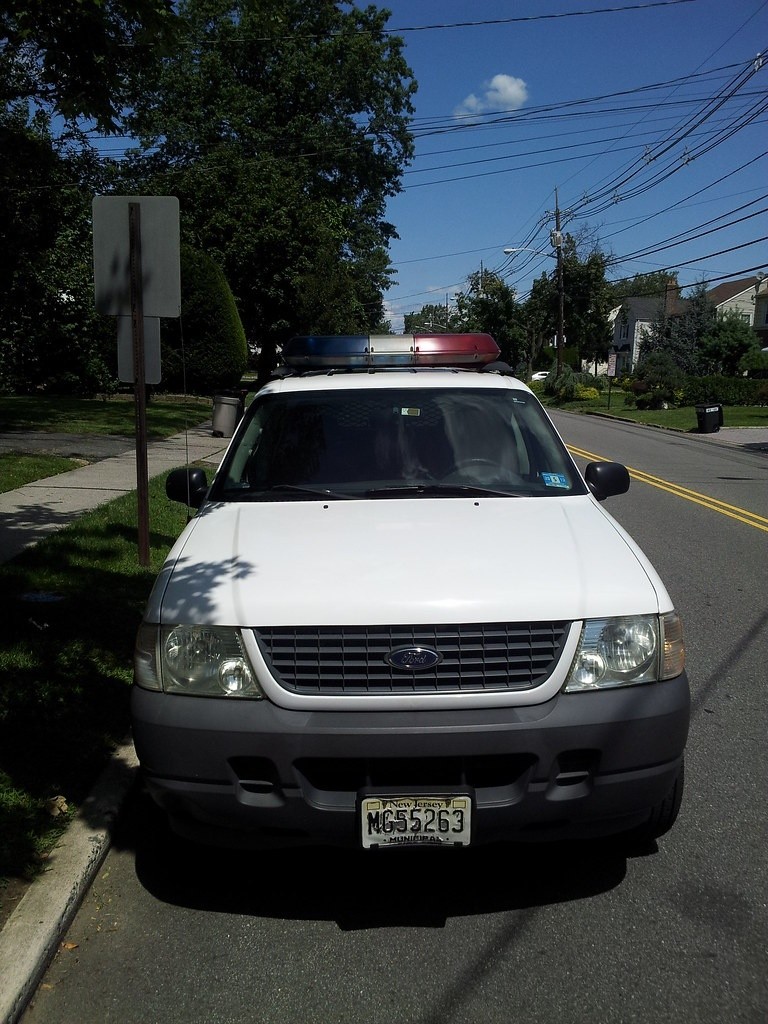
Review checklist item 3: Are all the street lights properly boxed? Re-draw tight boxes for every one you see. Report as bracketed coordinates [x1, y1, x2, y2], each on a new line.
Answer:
[450, 297, 484, 301]
[424, 322, 447, 331]
[415, 326, 432, 333]
[504, 248, 564, 378]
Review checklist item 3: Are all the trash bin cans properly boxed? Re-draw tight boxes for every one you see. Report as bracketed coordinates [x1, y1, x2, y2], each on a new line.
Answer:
[213, 388, 248, 433]
[695, 403, 723, 433]
[212, 395, 240, 438]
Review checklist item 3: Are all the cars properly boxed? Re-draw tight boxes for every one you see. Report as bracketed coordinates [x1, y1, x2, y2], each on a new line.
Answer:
[129, 331, 690, 854]
[532, 371, 550, 382]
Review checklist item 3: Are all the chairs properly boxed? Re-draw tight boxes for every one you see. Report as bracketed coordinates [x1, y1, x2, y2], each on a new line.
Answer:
[442, 407, 520, 476]
